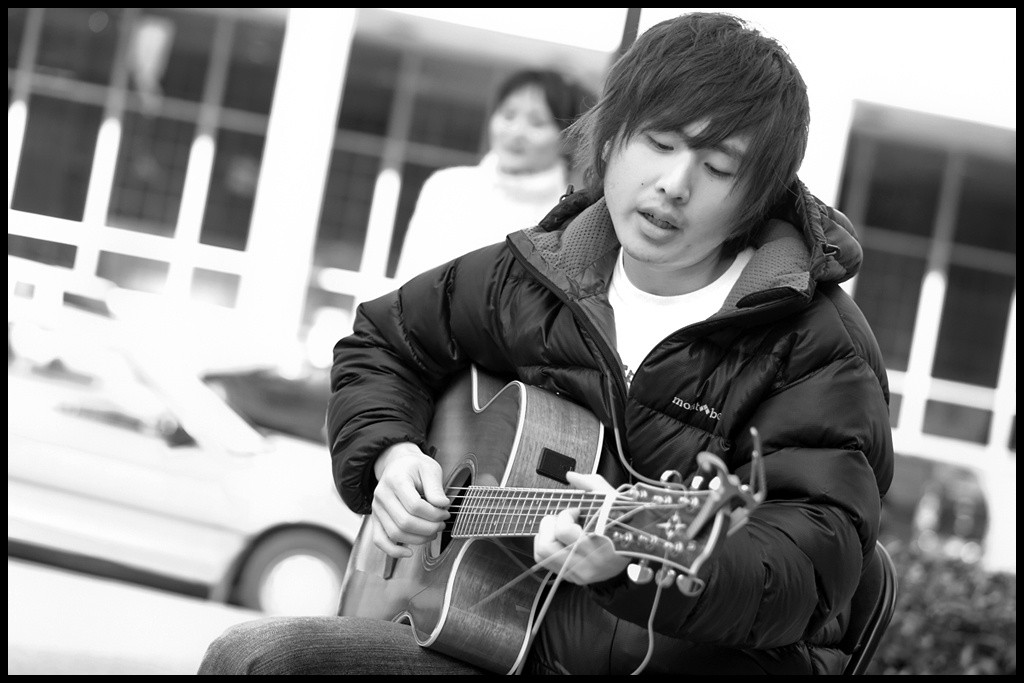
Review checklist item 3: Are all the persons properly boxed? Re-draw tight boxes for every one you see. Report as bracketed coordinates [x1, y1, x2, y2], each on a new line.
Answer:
[191, 9, 890, 674]
[393, 67, 593, 299]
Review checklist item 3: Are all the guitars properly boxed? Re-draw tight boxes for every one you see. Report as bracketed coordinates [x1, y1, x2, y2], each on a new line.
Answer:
[338, 365, 731, 675]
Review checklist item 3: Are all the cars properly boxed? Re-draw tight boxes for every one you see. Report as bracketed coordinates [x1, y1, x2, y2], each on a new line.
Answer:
[7, 259, 364, 621]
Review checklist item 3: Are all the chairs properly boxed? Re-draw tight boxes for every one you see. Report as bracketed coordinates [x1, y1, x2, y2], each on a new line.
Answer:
[839, 540, 900, 675]
